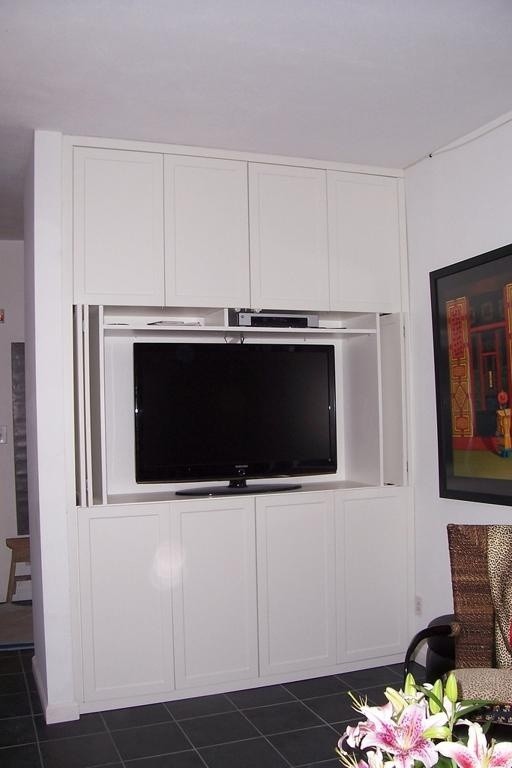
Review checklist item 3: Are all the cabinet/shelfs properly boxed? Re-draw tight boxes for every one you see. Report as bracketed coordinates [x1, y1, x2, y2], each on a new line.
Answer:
[25, 129, 418, 726]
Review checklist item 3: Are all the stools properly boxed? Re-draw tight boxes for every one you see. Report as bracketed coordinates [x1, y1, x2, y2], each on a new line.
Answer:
[5, 537, 31, 602]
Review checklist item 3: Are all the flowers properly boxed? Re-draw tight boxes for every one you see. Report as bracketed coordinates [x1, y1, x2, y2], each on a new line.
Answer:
[331, 672, 512, 768]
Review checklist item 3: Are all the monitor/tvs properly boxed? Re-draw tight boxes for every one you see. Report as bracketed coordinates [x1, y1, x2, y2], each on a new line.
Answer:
[133, 342, 337, 496]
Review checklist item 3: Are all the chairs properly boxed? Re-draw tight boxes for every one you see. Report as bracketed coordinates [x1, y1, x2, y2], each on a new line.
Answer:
[404, 524, 512, 742]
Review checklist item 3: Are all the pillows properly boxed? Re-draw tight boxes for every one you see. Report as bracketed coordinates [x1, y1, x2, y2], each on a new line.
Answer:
[439, 668, 512, 724]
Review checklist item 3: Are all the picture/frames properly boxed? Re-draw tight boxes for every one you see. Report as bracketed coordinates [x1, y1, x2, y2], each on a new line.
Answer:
[428, 243, 512, 507]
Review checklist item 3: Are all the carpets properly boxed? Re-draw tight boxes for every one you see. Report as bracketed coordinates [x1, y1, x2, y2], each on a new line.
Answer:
[0, 601, 34, 645]
